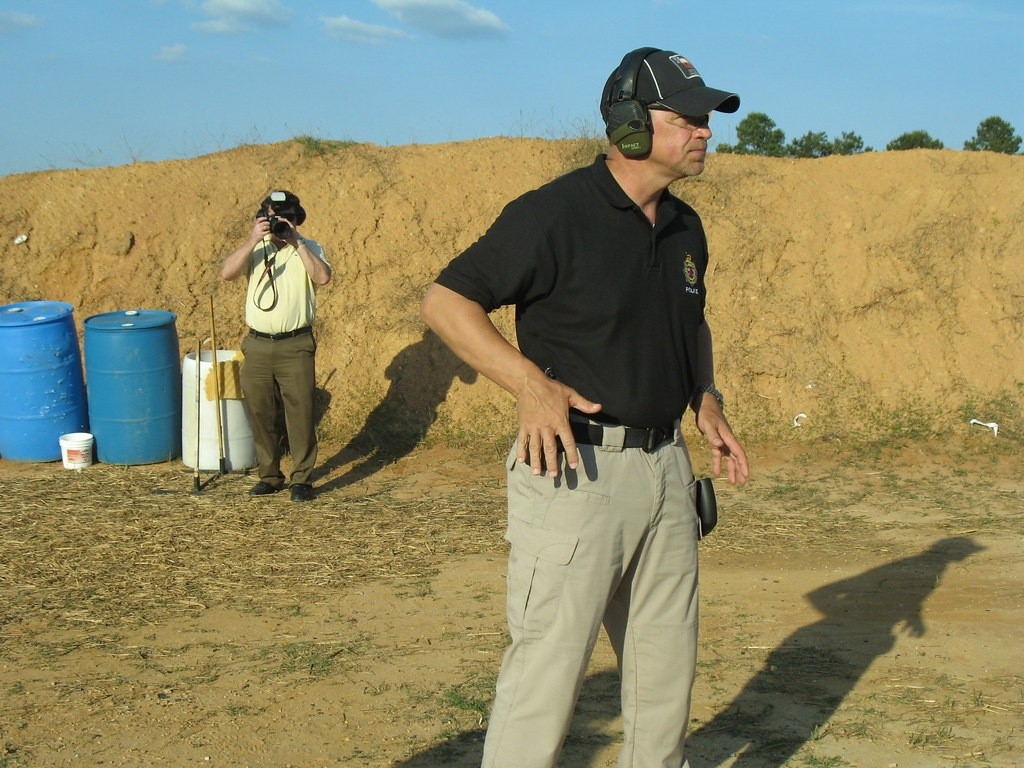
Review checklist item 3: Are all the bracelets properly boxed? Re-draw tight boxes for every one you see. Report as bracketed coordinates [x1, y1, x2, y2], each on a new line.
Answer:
[691, 385, 724, 410]
[295, 239, 304, 250]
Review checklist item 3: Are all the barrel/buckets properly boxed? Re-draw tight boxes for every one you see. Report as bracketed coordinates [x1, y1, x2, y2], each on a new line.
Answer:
[0, 301, 89, 462]
[58, 432, 94, 470]
[183, 350, 258, 471]
[82, 310, 181, 466]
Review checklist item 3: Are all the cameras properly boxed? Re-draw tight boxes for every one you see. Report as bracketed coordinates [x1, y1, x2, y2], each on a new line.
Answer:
[264, 214, 292, 239]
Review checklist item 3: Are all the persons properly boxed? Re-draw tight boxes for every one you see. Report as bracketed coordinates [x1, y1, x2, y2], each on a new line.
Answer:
[222, 191, 331, 502]
[420, 48, 750, 768]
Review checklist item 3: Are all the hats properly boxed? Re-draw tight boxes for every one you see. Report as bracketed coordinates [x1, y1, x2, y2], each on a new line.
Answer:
[600, 49, 740, 118]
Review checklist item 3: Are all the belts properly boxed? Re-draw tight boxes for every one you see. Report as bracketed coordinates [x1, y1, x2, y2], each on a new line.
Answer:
[565, 420, 674, 453]
[249, 328, 312, 340]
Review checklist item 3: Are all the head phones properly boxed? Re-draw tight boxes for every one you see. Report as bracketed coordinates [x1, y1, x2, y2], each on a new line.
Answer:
[256, 190, 306, 226]
[606, 46, 662, 158]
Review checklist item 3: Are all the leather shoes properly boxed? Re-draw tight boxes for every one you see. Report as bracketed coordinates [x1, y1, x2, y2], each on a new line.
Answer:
[291, 483, 312, 502]
[249, 480, 275, 494]
